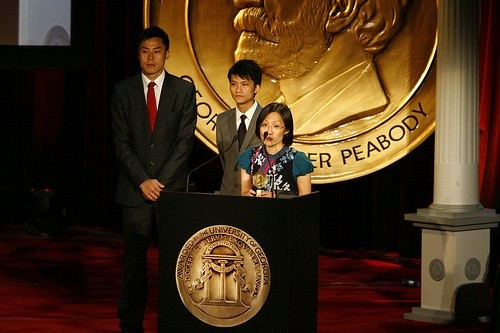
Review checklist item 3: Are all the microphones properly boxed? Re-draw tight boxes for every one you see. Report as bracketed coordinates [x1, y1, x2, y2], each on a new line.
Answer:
[186, 135, 239, 192]
[263, 131, 274, 198]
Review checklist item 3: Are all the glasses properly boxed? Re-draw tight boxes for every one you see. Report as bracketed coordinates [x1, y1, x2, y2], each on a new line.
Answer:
[260, 124, 285, 130]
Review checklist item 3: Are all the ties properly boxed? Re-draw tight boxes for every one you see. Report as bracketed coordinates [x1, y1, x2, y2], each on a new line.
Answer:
[146, 82, 157, 134]
[237, 114, 247, 152]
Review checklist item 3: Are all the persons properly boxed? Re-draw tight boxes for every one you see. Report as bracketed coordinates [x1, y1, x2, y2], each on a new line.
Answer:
[112, 26, 197, 333]
[216, 59, 264, 196]
[238, 102, 314, 198]
